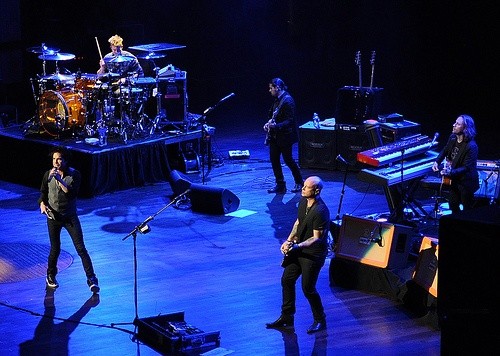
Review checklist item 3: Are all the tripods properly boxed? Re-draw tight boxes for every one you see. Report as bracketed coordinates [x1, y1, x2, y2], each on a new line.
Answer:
[20, 52, 185, 147]
[110, 200, 175, 356]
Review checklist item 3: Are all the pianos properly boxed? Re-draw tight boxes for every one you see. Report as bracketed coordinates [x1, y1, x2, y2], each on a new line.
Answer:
[356, 133, 446, 223]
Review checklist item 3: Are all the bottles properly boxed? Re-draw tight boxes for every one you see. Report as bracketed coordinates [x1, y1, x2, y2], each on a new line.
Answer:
[313, 114, 320, 129]
[99, 128, 107, 147]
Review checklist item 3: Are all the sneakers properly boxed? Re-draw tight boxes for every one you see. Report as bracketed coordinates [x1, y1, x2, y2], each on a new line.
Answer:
[46, 274, 59, 288]
[90, 284, 100, 294]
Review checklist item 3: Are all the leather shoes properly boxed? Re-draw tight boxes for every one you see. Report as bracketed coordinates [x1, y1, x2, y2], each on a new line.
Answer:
[290, 182, 302, 193]
[265, 315, 294, 327]
[267, 185, 287, 193]
[306, 322, 326, 333]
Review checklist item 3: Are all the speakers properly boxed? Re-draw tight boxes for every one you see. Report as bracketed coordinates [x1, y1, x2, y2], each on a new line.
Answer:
[156, 71, 186, 125]
[337, 133, 367, 172]
[190, 183, 240, 215]
[335, 214, 413, 269]
[299, 121, 336, 168]
[411, 236, 438, 299]
[168, 170, 195, 196]
[438, 203, 500, 356]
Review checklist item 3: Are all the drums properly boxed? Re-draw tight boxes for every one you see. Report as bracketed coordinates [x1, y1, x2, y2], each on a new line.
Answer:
[38, 73, 145, 137]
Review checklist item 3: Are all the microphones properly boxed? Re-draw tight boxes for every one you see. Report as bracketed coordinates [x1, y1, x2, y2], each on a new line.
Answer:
[216, 92, 236, 105]
[47, 167, 57, 183]
[427, 132, 440, 150]
[335, 154, 352, 170]
[174, 188, 192, 201]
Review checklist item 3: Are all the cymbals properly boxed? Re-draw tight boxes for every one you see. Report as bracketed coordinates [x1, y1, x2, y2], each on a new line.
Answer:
[103, 56, 137, 63]
[43, 73, 74, 80]
[26, 46, 76, 61]
[135, 52, 167, 60]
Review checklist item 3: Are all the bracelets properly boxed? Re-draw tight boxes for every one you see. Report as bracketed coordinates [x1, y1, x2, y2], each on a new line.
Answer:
[287, 240, 293, 242]
[100, 68, 105, 71]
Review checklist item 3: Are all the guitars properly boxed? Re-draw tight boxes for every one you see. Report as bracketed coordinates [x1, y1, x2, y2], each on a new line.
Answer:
[354, 49, 377, 125]
[439, 146, 459, 199]
[263, 107, 281, 146]
[280, 233, 300, 268]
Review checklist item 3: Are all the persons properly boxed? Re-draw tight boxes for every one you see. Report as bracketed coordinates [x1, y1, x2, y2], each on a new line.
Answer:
[38, 147, 100, 293]
[97, 35, 144, 78]
[265, 176, 330, 333]
[432, 115, 480, 213]
[263, 78, 304, 193]
